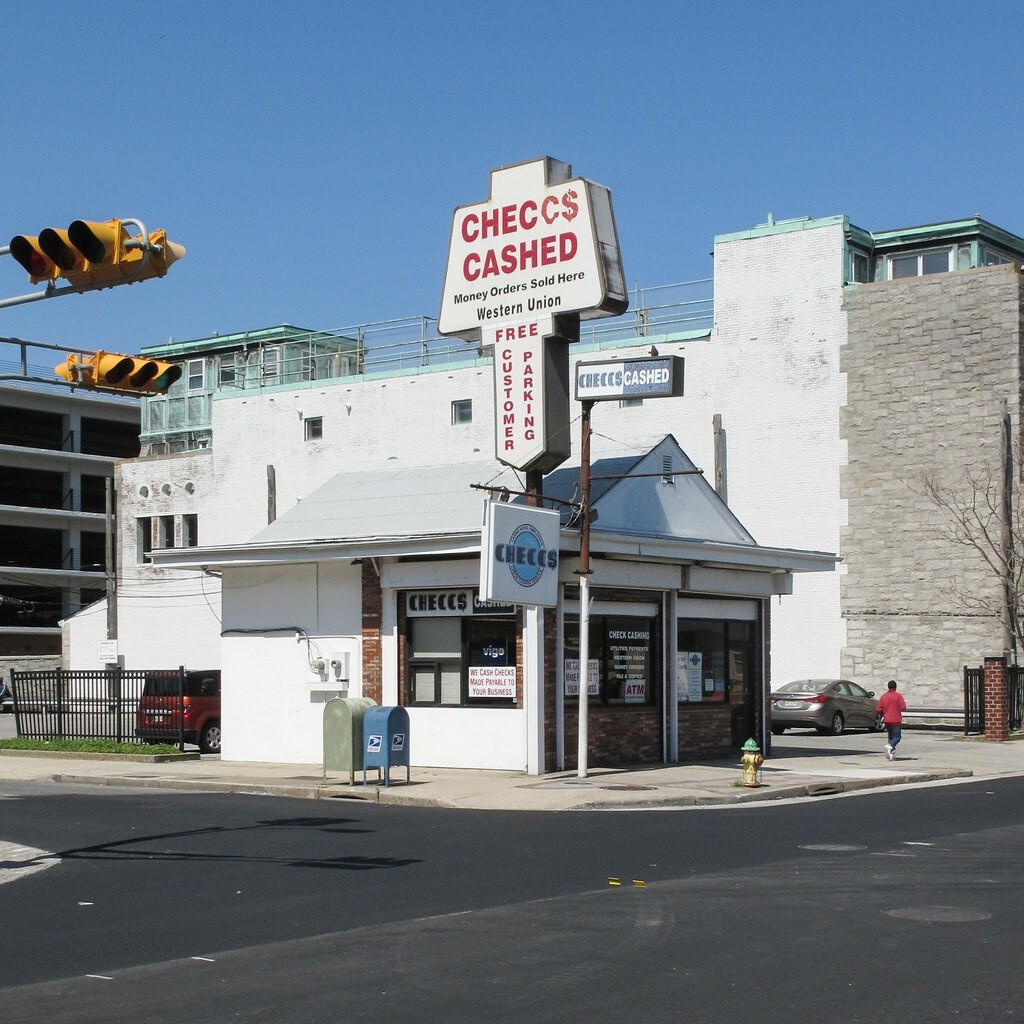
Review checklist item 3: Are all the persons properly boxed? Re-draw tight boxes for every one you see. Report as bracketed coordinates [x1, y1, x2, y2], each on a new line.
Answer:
[876, 681, 906, 760]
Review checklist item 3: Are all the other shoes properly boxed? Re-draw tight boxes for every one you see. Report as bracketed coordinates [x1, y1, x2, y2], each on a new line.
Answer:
[884, 743, 897, 761]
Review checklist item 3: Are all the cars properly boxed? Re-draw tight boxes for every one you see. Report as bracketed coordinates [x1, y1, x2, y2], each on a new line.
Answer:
[769, 679, 888, 737]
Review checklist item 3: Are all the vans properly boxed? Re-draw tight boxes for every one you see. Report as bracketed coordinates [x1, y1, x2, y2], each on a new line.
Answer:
[135, 667, 221, 754]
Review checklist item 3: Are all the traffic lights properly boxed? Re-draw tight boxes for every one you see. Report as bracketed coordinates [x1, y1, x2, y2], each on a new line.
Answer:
[53, 348, 183, 400]
[9, 219, 133, 286]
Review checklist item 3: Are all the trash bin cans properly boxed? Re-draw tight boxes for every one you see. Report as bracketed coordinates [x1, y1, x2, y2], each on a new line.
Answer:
[322, 697, 379, 786]
[362, 705, 410, 787]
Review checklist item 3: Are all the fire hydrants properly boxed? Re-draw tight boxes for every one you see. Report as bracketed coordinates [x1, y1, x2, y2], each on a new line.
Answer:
[740, 737, 764, 787]
